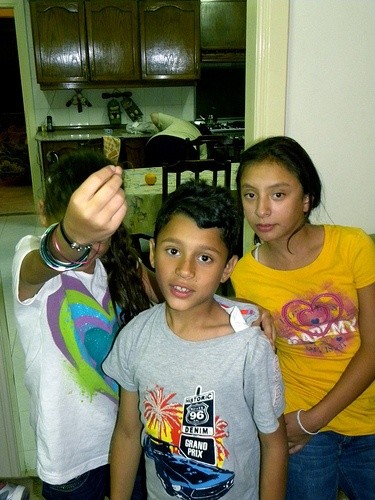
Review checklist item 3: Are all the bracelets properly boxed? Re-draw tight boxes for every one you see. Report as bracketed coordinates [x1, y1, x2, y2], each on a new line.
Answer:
[296, 409, 320, 435]
[39, 219, 101, 272]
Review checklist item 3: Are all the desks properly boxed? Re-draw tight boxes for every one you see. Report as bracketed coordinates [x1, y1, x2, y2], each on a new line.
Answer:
[122, 163, 241, 238]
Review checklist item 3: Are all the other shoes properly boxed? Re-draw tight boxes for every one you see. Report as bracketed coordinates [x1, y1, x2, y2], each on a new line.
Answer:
[0, 481, 30, 500]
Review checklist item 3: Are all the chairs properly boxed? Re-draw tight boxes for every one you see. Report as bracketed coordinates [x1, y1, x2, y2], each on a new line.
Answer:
[162, 135, 241, 204]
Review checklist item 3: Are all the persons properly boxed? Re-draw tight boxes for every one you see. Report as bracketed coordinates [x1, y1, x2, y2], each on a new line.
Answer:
[10, 152, 277, 500]
[147, 111, 208, 160]
[230, 136, 375, 500]
[101, 179, 289, 500]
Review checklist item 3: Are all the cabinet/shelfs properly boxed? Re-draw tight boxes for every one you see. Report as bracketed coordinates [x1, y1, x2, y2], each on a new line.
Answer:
[29, 0, 246, 92]
[35, 120, 244, 197]
